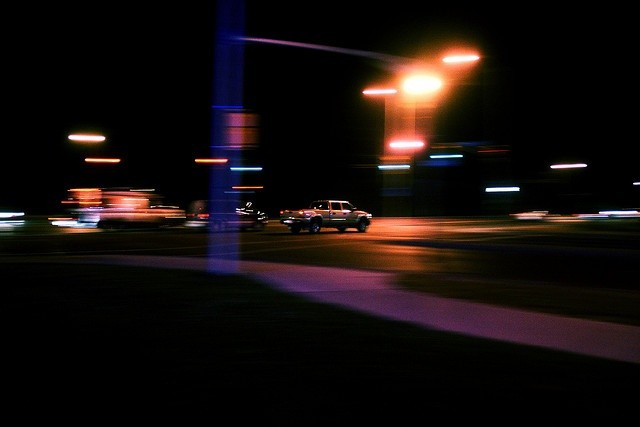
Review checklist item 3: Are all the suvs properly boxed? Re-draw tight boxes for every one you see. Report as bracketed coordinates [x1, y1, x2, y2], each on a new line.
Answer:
[185, 199, 268, 232]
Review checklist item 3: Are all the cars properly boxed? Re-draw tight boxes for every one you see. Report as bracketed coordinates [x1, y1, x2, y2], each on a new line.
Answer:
[281, 200, 371, 234]
